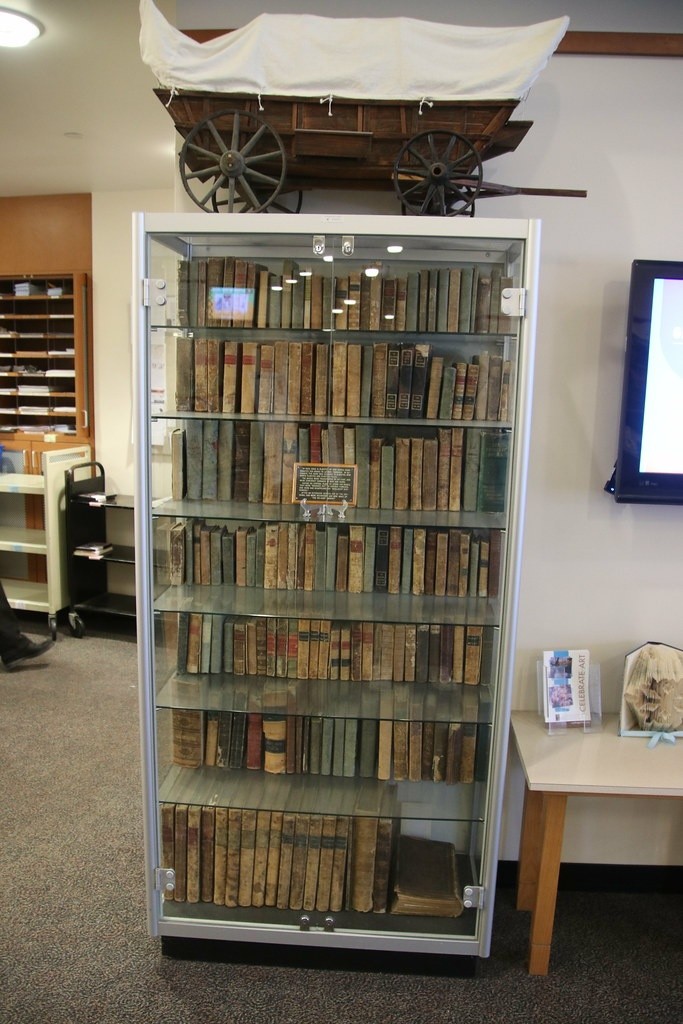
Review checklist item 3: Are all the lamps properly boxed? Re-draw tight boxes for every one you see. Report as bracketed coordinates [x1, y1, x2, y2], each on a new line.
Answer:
[0, 4, 45, 49]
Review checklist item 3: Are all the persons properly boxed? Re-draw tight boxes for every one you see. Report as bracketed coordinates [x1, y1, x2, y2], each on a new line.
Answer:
[0, 580, 55, 672]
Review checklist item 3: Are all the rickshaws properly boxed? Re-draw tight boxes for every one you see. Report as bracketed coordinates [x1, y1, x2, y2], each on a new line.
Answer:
[150, 80, 587, 220]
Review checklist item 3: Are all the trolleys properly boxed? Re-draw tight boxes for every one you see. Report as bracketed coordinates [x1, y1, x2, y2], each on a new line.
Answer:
[0, 442, 95, 643]
[63, 460, 185, 639]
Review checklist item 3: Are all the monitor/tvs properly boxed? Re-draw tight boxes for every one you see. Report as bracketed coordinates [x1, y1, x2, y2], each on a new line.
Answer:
[603, 259, 682, 504]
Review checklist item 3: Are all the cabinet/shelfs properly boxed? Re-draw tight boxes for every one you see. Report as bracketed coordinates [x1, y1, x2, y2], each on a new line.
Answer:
[1, 442, 94, 640]
[66, 462, 161, 640]
[0, 267, 88, 451]
[130, 206, 544, 958]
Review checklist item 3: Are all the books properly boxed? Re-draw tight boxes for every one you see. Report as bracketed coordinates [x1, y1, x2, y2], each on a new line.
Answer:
[175, 597, 495, 689]
[167, 518, 501, 599]
[174, 336, 517, 420]
[177, 258, 515, 332]
[168, 674, 494, 786]
[156, 765, 464, 917]
[168, 418, 512, 513]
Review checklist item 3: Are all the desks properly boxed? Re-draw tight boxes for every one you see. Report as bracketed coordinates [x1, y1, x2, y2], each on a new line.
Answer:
[512, 704, 683, 982]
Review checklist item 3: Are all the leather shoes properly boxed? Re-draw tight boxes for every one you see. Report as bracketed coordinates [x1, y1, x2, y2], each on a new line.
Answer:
[4, 635, 57, 667]
